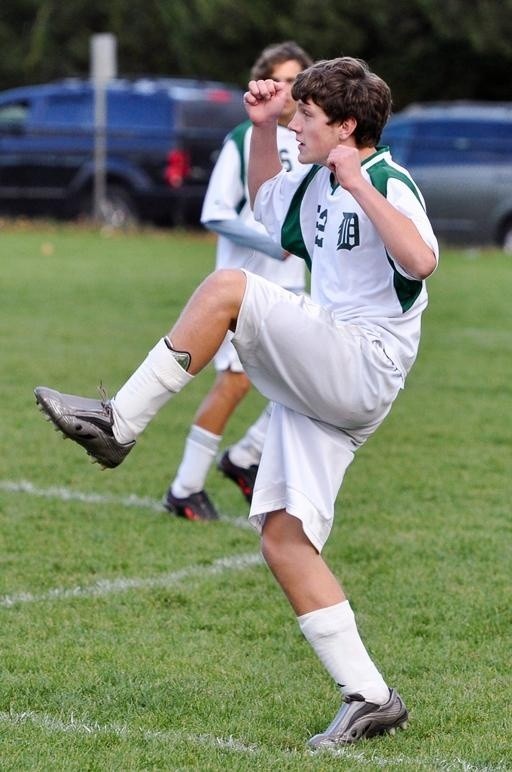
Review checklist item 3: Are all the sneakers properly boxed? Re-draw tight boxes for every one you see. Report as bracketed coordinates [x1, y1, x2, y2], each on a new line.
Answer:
[160, 485, 219, 520]
[216, 446, 258, 506]
[308, 688, 408, 749]
[34, 379, 136, 471]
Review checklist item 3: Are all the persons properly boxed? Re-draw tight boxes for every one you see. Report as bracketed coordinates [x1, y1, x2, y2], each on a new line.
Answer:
[34, 57, 443, 754]
[161, 41, 317, 527]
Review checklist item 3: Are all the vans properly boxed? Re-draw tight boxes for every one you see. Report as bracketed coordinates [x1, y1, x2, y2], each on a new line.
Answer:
[377, 107, 511, 251]
[0, 80, 248, 227]
